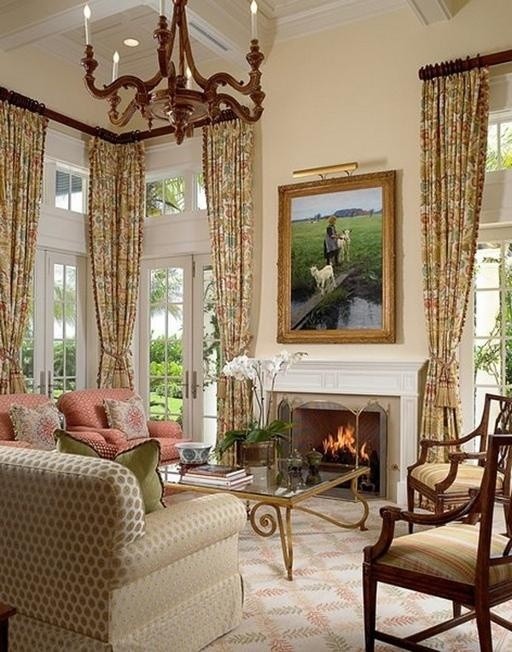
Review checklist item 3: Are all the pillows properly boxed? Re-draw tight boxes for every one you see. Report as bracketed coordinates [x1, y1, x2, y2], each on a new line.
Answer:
[104, 395, 151, 439]
[9, 402, 59, 450]
[53, 430, 166, 514]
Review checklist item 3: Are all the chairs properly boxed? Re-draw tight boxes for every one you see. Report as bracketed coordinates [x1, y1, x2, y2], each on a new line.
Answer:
[404, 393, 511, 539]
[61, 387, 188, 480]
[0, 394, 105, 455]
[360, 434, 511, 652]
[1, 446, 250, 651]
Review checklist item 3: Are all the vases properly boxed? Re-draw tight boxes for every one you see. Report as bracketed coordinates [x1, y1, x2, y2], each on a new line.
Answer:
[243, 439, 278, 469]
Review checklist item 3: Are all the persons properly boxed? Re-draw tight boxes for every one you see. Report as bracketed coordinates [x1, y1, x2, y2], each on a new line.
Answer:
[324, 216, 341, 266]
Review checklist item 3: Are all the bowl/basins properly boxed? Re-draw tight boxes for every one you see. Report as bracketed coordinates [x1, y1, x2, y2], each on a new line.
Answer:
[174, 442, 213, 465]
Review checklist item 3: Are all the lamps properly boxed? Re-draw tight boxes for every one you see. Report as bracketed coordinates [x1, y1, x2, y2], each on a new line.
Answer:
[81, 2, 265, 143]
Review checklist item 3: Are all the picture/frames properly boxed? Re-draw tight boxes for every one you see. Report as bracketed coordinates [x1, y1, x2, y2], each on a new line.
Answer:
[277, 170, 398, 343]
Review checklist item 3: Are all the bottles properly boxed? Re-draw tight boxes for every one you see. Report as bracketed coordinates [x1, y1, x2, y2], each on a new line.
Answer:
[286, 471, 306, 493]
[305, 448, 323, 473]
[286, 449, 304, 472]
[305, 471, 322, 487]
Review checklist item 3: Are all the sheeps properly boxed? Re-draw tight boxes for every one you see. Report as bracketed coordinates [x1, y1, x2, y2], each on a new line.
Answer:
[336, 228, 353, 264]
[309, 261, 336, 296]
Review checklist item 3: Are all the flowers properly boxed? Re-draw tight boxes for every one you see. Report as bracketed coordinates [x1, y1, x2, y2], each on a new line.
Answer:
[212, 353, 303, 457]
